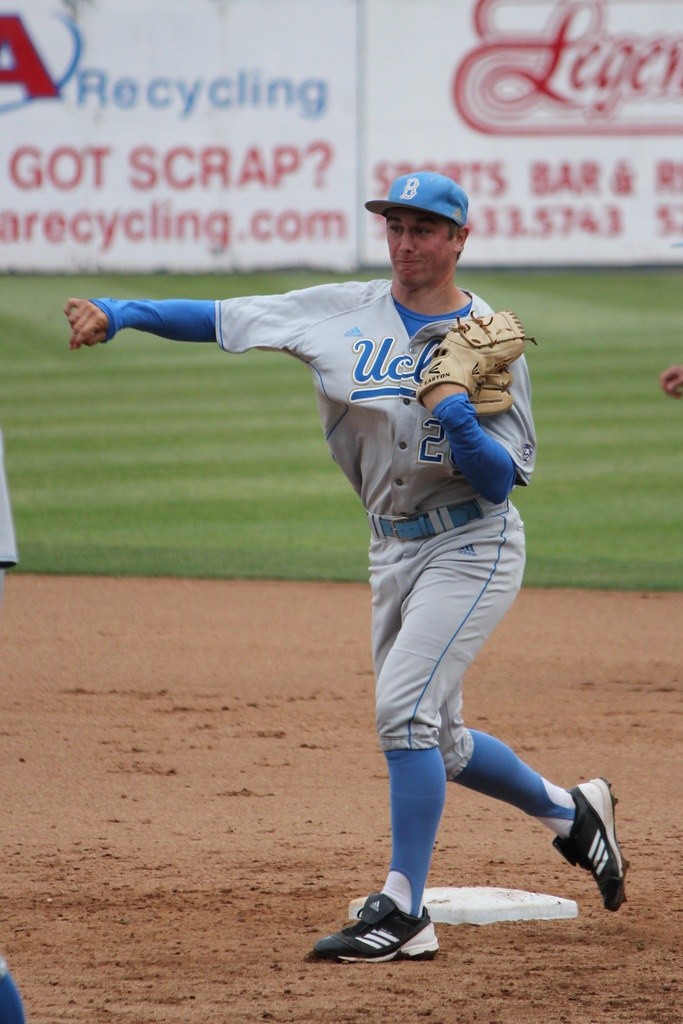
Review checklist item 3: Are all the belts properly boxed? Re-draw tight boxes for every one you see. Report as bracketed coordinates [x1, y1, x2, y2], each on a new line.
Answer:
[368, 498, 481, 539]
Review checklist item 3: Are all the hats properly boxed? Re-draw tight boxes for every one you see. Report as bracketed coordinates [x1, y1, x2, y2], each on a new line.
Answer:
[365, 171, 468, 227]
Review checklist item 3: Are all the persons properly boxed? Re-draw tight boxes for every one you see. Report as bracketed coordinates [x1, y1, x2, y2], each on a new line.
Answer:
[61, 171, 633, 963]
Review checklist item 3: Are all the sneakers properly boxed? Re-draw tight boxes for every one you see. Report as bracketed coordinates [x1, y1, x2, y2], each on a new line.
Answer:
[552, 778, 624, 912]
[313, 891, 439, 962]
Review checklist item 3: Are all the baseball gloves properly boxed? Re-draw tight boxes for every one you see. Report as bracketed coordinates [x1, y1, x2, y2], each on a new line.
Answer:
[415, 309, 528, 418]
